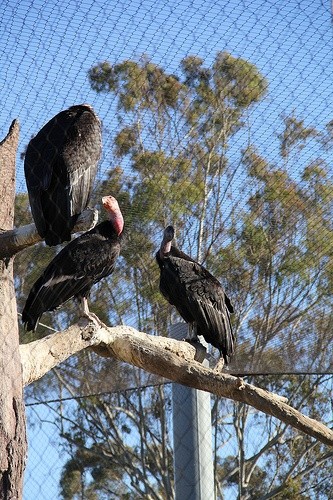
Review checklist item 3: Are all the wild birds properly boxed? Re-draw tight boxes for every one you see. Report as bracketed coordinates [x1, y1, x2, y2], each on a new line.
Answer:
[20, 195, 121, 335]
[22, 101, 102, 247]
[153, 223, 242, 367]
